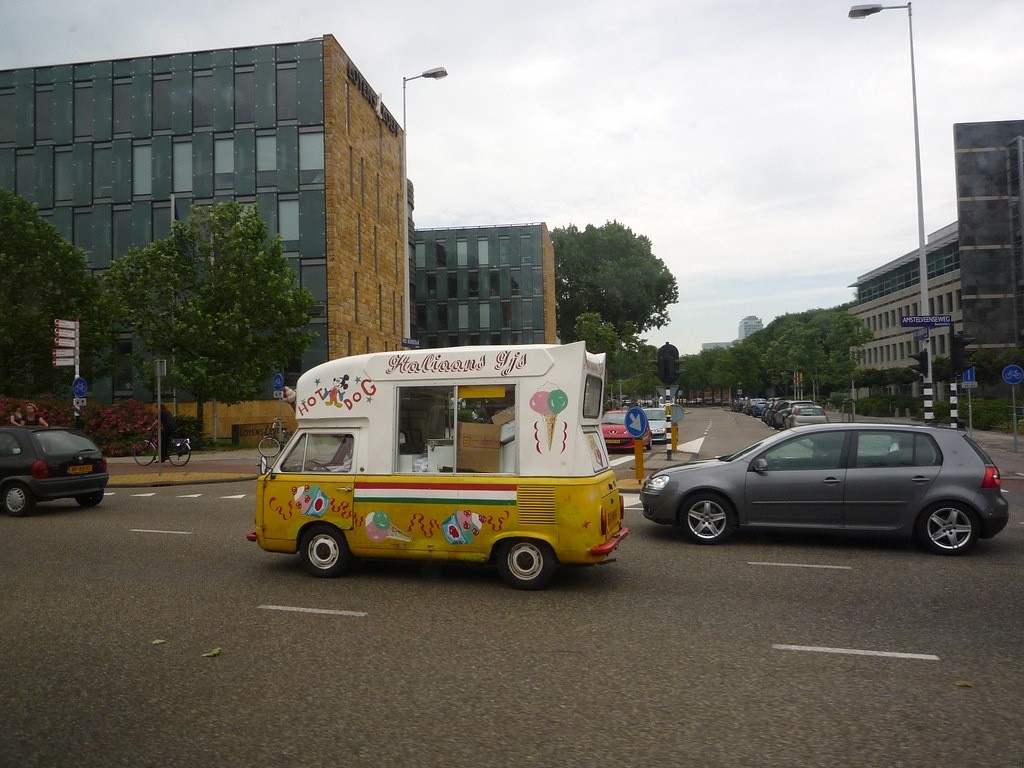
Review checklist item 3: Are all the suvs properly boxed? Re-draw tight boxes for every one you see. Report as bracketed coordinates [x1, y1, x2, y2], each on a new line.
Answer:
[771, 398, 815, 429]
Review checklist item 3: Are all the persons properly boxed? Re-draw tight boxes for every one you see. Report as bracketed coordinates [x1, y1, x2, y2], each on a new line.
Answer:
[20, 402, 49, 428]
[10, 404, 26, 428]
[843, 397, 855, 422]
[143, 403, 176, 463]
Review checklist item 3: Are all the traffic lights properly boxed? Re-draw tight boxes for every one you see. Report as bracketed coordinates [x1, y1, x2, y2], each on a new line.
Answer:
[952, 335, 977, 376]
[648, 355, 666, 380]
[907, 348, 928, 378]
[668, 355, 686, 384]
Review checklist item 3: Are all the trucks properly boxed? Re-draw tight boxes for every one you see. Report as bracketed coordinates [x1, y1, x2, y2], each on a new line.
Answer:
[246, 339, 629, 591]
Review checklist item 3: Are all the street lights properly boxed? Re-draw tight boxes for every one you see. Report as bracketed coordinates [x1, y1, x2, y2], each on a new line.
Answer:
[846, 2, 935, 426]
[401, 66, 450, 349]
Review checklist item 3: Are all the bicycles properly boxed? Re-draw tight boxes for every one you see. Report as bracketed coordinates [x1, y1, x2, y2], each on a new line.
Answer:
[132, 426, 191, 467]
[257, 416, 293, 458]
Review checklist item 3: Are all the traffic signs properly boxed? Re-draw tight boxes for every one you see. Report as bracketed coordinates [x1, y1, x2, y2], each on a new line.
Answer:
[53, 337, 75, 348]
[53, 328, 75, 338]
[51, 358, 74, 368]
[54, 319, 76, 330]
[51, 348, 75, 358]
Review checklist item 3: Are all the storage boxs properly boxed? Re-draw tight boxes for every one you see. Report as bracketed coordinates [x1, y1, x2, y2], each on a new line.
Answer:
[396, 404, 517, 474]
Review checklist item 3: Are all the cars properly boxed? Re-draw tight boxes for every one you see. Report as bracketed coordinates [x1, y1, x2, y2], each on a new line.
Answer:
[641, 408, 666, 442]
[0, 421, 108, 518]
[600, 410, 653, 452]
[783, 404, 829, 431]
[603, 393, 776, 426]
[639, 421, 1009, 558]
